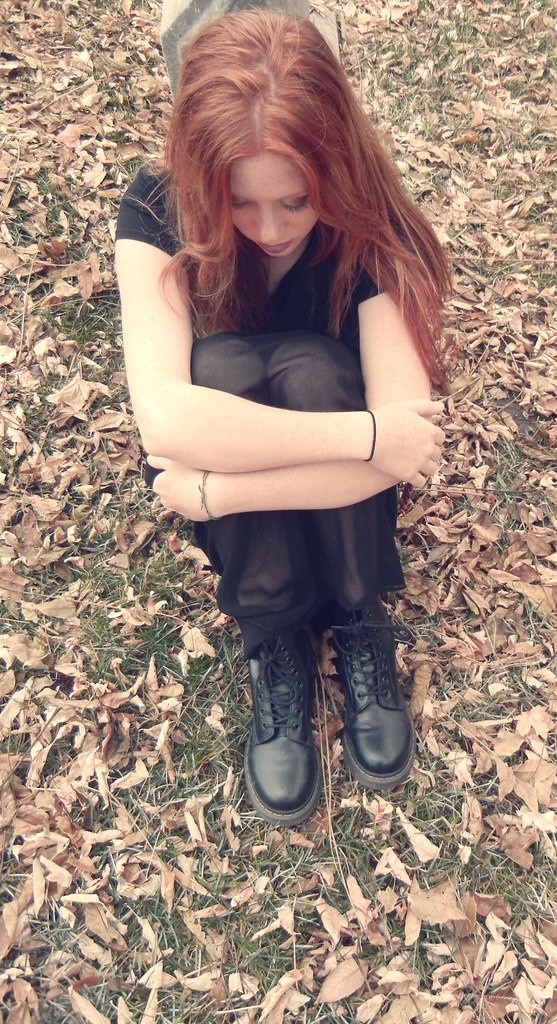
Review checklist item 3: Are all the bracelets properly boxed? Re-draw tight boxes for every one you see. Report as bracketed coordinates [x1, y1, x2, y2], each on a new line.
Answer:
[199, 472, 218, 524]
[364, 408, 376, 463]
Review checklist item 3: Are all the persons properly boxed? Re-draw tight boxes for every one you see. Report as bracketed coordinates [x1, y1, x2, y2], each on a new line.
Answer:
[115, 12, 452, 826]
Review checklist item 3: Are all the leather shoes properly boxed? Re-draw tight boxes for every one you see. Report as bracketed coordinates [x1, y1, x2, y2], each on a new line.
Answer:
[328, 601, 415, 787]
[244, 633, 321, 826]
